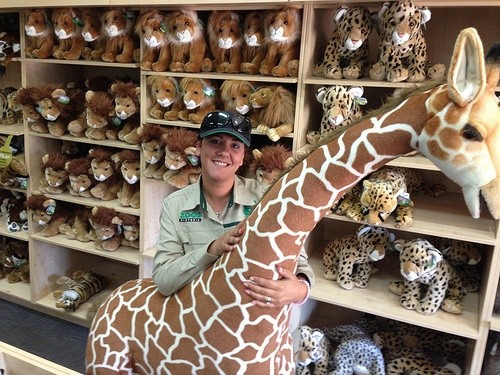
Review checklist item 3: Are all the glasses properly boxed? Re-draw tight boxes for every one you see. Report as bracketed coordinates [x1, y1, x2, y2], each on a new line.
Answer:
[199, 112, 253, 134]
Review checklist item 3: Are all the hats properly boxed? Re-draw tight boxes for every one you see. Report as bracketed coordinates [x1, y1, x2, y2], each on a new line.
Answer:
[198, 110, 251, 146]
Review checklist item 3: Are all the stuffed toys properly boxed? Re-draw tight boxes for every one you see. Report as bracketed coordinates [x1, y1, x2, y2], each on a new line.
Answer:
[0, 0, 500, 375]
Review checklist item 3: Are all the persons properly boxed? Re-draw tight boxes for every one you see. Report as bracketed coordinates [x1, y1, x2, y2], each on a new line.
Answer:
[152, 109, 314, 357]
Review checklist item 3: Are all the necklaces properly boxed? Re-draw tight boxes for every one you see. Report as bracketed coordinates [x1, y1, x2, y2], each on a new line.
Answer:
[209, 202, 221, 218]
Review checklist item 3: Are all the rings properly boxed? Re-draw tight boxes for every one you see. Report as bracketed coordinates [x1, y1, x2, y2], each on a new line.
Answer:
[266, 297, 272, 302]
[267, 302, 271, 305]
